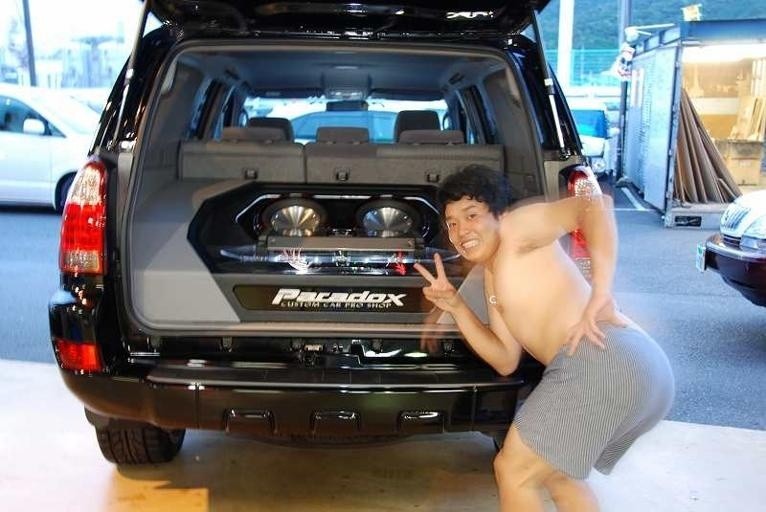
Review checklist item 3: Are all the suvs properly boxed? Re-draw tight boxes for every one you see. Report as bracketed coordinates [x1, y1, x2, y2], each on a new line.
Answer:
[45, 0, 602, 466]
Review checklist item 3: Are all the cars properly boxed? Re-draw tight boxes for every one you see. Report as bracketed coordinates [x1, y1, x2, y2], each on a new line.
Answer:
[556, 95, 630, 180]
[62, 86, 115, 119]
[690, 189, 766, 309]
[0, 83, 103, 217]
[263, 107, 454, 148]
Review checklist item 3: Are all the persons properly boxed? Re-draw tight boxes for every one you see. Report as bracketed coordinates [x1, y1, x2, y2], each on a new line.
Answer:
[411, 163, 676, 512]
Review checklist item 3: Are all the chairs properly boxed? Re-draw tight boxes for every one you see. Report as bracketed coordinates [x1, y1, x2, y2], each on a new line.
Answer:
[222, 108, 471, 145]
